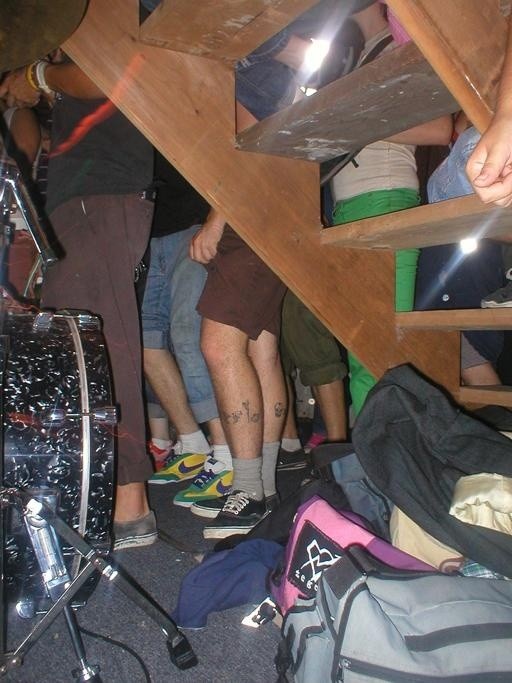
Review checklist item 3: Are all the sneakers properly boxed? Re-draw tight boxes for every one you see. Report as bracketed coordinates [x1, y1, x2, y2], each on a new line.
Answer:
[146, 432, 327, 542]
[110, 510, 157, 553]
[480, 281, 512, 308]
[316, 27, 398, 92]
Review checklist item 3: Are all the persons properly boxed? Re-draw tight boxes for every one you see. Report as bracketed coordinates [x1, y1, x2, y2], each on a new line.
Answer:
[0, 0, 512, 552]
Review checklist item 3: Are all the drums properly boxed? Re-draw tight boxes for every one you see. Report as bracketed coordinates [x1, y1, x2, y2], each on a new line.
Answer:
[1, 307, 118, 618]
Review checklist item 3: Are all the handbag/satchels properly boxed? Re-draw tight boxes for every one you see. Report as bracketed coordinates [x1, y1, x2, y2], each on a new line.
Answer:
[268, 494, 512, 683]
